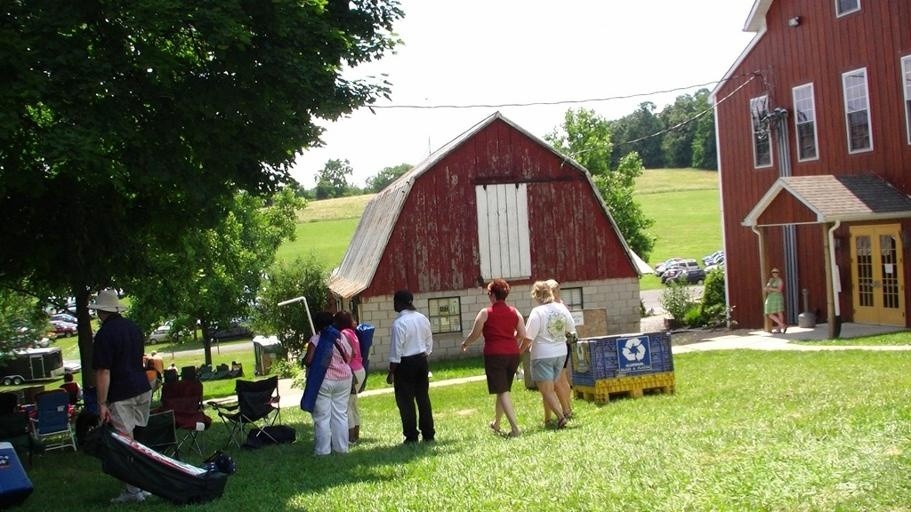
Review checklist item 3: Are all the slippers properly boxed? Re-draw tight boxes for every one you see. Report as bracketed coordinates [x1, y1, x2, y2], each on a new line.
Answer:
[487, 420, 524, 438]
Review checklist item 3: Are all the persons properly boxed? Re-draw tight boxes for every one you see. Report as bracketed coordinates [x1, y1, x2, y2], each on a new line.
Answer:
[59, 373, 81, 400]
[86, 290, 153, 503]
[519, 280, 575, 428]
[335, 310, 361, 445]
[546, 278, 579, 418]
[763, 268, 789, 334]
[387, 290, 435, 444]
[167, 362, 177, 372]
[151, 350, 163, 368]
[460, 278, 528, 436]
[301, 310, 353, 455]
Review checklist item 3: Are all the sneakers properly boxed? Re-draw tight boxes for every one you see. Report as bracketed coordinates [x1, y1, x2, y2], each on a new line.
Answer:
[771, 324, 790, 335]
[108, 486, 154, 504]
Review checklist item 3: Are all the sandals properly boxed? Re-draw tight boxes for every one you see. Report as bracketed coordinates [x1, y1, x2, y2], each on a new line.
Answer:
[541, 410, 575, 430]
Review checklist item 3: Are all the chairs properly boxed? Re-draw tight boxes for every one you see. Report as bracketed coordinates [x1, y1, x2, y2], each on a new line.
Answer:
[182, 366, 198, 381]
[133, 409, 178, 460]
[28, 388, 77, 456]
[163, 369, 179, 382]
[207, 375, 282, 449]
[159, 381, 213, 458]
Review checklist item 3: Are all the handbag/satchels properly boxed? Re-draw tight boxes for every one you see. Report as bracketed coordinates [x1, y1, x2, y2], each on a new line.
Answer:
[565, 331, 580, 345]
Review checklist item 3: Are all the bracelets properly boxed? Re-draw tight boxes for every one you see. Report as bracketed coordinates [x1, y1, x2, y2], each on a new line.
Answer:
[462, 342, 467, 347]
[98, 401, 108, 406]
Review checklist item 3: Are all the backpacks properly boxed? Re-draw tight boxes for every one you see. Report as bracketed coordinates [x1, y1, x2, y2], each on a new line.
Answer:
[298, 324, 341, 413]
[355, 321, 376, 394]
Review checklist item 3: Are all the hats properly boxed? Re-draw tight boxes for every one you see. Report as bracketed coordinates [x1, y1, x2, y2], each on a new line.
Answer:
[393, 288, 416, 311]
[545, 279, 561, 290]
[86, 290, 130, 314]
[770, 267, 781, 272]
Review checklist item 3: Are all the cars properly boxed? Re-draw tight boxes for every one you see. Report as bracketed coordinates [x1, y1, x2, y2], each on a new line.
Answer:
[142, 315, 256, 345]
[655, 251, 725, 289]
[1, 289, 124, 352]
